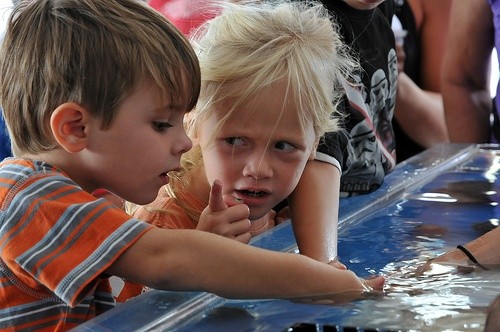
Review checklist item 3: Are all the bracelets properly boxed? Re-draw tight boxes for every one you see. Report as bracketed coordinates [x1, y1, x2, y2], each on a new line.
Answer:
[456, 242, 491, 273]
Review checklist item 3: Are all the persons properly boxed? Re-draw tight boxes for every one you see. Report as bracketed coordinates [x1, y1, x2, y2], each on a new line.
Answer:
[439, 0, 499, 144]
[115, 3, 345, 305]
[393, 2, 454, 149]
[300, 0, 404, 293]
[0, 0, 389, 332]
[411, 222, 500, 288]
